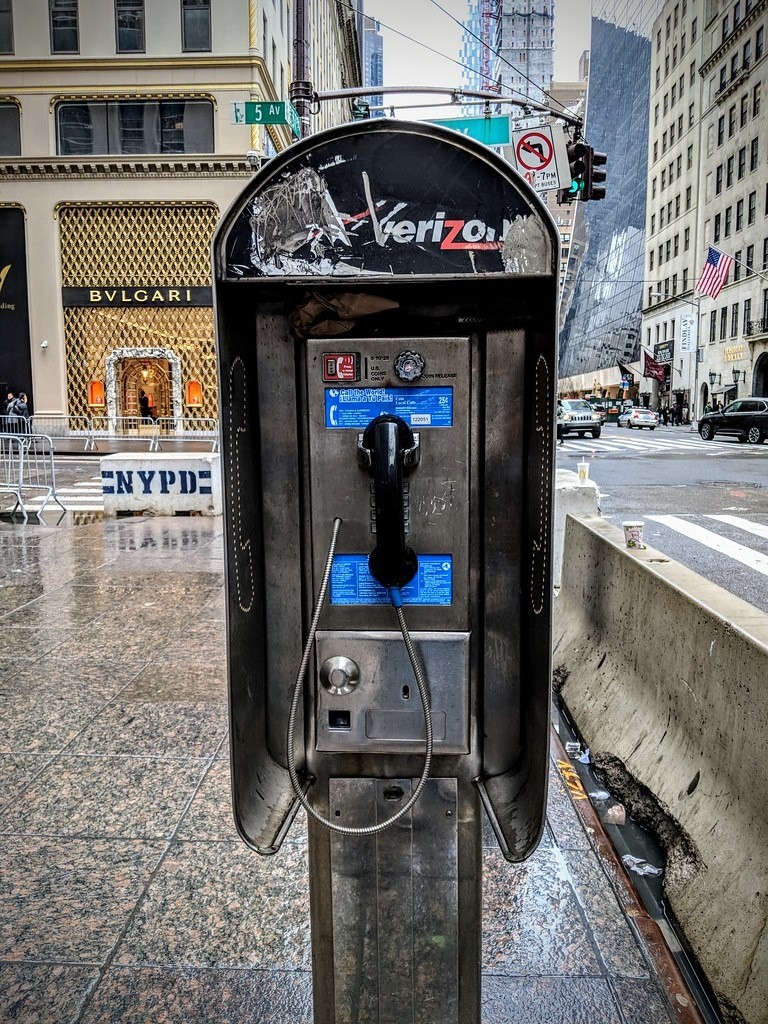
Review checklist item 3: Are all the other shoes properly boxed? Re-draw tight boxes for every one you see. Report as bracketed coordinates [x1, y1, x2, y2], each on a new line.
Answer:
[559, 442, 564, 446]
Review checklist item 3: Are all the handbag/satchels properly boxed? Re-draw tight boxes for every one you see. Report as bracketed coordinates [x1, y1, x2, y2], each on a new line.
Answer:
[13, 401, 26, 415]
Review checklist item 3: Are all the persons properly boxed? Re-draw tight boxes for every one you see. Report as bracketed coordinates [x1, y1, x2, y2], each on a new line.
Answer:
[713, 400, 724, 412]
[3, 392, 39, 444]
[654, 398, 689, 427]
[556, 400, 567, 446]
[704, 401, 713, 417]
[138, 390, 149, 425]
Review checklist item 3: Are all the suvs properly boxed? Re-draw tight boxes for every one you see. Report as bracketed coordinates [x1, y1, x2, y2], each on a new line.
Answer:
[698, 397, 768, 445]
[589, 404, 606, 426]
[555, 398, 604, 439]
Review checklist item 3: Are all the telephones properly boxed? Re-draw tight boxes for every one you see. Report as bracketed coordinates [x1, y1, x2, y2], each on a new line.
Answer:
[305, 334, 474, 631]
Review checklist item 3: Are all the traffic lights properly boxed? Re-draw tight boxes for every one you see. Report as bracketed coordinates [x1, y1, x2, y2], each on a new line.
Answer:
[579, 144, 607, 202]
[649, 292, 699, 431]
[558, 142, 588, 206]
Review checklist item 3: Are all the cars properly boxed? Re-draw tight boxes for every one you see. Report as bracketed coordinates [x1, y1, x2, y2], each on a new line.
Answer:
[616, 408, 658, 430]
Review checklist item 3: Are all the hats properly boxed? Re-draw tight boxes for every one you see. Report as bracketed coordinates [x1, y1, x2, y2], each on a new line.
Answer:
[17, 392, 24, 398]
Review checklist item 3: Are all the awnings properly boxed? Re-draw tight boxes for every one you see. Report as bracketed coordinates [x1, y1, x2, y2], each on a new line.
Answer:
[711, 385, 737, 395]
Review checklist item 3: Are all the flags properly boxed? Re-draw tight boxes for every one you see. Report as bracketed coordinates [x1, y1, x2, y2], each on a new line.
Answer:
[617, 359, 635, 387]
[693, 246, 733, 300]
[643, 351, 664, 383]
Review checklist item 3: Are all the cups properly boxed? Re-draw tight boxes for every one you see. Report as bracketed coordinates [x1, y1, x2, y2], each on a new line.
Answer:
[577, 463, 589, 483]
[622, 521, 644, 549]
